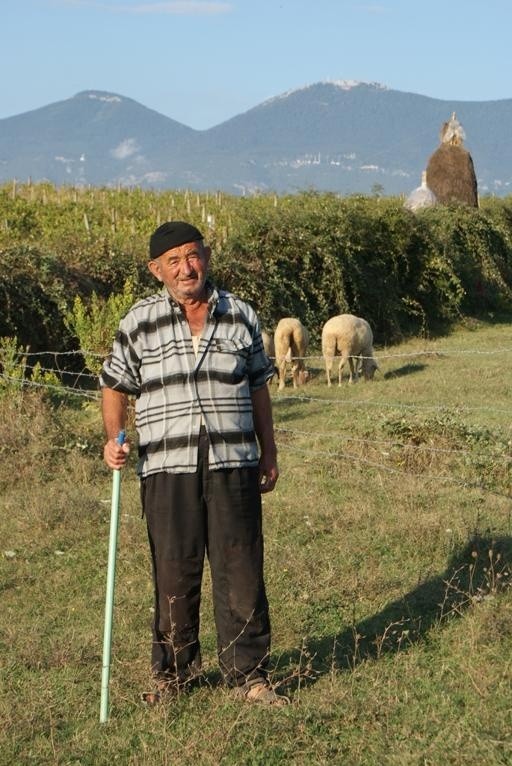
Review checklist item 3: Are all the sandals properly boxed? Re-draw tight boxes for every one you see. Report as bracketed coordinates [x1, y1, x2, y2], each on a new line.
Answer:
[231, 678, 285, 707]
[142, 674, 190, 704]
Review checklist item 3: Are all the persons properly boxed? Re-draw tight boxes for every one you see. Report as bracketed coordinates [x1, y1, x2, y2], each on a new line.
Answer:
[95, 217, 290, 712]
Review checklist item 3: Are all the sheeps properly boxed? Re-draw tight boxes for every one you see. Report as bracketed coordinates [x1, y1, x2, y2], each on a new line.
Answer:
[261, 317, 311, 390]
[321, 314, 382, 389]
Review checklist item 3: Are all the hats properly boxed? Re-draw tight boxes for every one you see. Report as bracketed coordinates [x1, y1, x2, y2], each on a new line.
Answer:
[150, 221, 204, 259]
